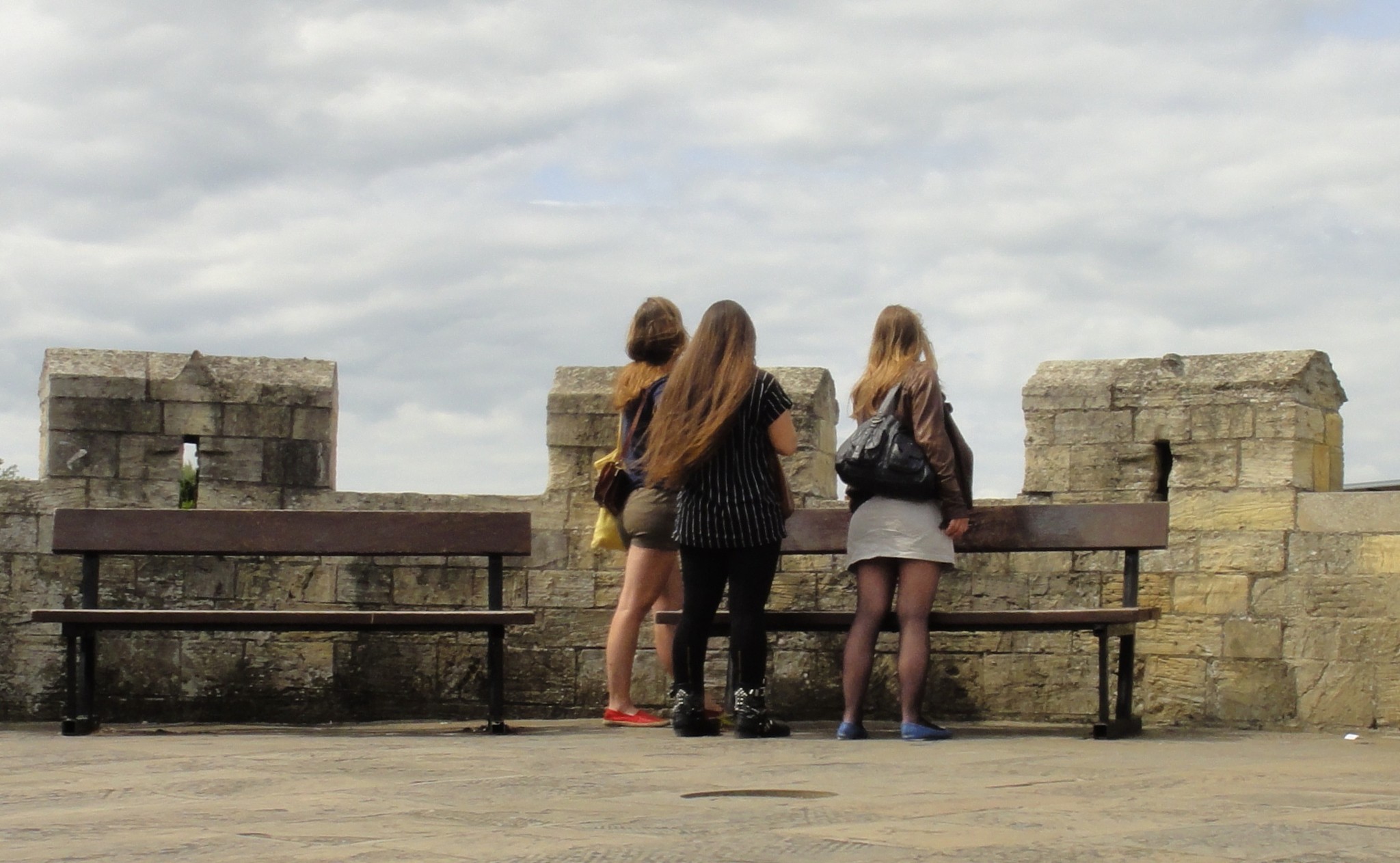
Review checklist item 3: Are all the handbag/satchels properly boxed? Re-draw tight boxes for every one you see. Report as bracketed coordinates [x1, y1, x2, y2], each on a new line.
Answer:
[589, 449, 634, 554]
[592, 461, 634, 516]
[834, 372, 937, 502]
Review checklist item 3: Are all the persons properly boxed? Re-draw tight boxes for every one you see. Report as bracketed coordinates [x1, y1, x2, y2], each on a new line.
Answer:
[603, 298, 728, 728]
[837, 305, 971, 742]
[641, 301, 797, 740]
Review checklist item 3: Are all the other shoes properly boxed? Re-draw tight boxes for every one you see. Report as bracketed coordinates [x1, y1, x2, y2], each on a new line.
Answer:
[836, 722, 870, 741]
[602, 709, 670, 727]
[901, 721, 953, 741]
[703, 691, 723, 715]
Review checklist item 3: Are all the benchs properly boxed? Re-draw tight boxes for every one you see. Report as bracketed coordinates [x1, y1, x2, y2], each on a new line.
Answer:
[28, 509, 533, 735]
[654, 502, 1173, 740]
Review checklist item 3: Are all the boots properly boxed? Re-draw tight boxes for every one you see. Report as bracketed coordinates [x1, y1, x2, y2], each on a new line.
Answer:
[670, 690, 723, 737]
[734, 687, 791, 740]
